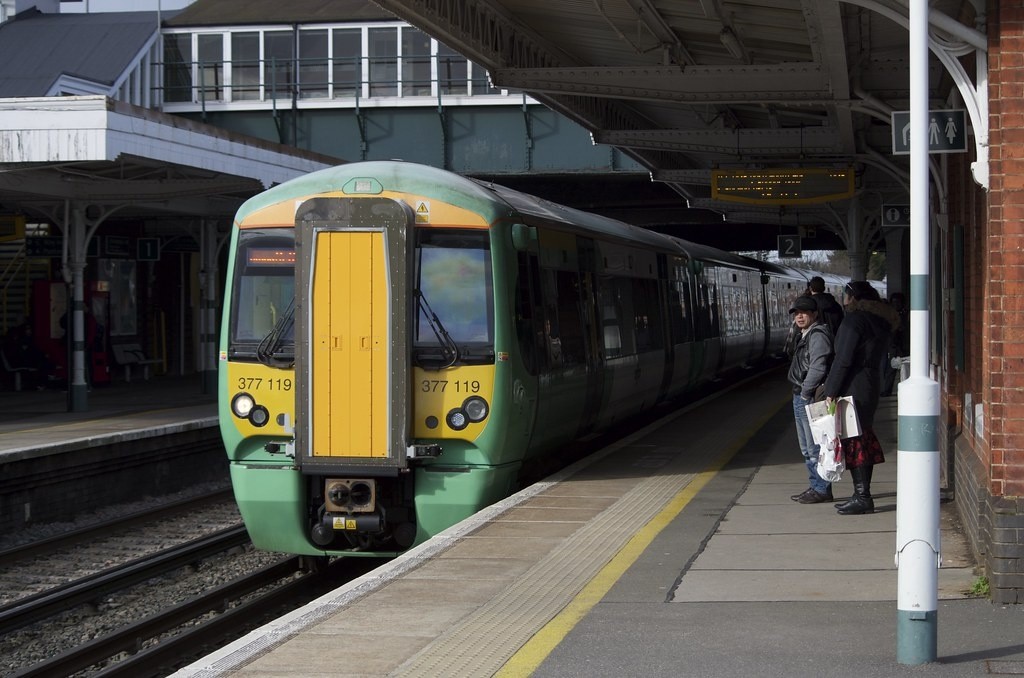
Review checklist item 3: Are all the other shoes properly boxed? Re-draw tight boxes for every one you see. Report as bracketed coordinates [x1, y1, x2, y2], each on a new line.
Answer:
[881, 391, 892, 396]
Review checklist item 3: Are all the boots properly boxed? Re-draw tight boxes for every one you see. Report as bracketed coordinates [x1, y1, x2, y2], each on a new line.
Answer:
[835, 464, 874, 508]
[837, 467, 874, 514]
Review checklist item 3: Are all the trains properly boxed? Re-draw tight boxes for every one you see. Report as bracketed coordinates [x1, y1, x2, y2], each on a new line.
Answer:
[218, 160, 887, 557]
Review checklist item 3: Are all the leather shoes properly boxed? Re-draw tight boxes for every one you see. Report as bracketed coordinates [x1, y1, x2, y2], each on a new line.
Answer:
[790, 487, 813, 501]
[798, 488, 832, 504]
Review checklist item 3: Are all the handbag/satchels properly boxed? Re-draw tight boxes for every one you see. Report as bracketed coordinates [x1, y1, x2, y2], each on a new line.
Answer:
[840, 419, 885, 470]
[816, 404, 845, 482]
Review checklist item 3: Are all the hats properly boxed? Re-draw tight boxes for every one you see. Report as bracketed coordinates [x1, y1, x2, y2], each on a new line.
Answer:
[789, 296, 818, 314]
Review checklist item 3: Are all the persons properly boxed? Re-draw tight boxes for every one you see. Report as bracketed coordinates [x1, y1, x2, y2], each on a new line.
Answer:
[786, 276, 908, 516]
